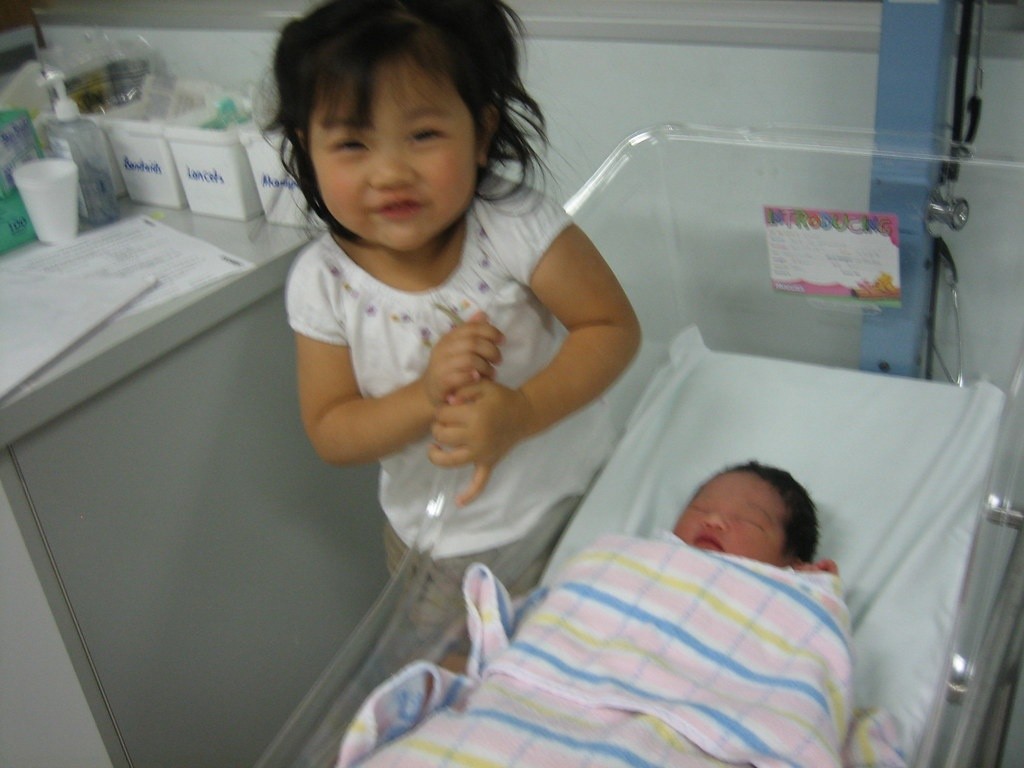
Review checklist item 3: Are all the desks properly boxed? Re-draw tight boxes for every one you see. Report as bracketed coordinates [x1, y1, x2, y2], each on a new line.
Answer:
[0, 203, 387, 768]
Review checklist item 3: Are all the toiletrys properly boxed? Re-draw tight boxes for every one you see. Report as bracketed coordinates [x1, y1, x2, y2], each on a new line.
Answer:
[28, 69, 122, 227]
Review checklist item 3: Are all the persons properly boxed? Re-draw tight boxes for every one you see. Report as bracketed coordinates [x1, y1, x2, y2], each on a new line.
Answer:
[337, 459, 851, 768]
[255, 0, 640, 677]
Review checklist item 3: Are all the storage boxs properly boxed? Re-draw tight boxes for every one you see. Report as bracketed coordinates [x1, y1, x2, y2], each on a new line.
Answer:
[239, 118, 331, 232]
[164, 116, 263, 221]
[100, 113, 188, 211]
[37, 100, 141, 199]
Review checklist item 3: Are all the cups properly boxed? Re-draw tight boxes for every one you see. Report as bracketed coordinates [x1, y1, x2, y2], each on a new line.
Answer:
[13, 159, 79, 244]
